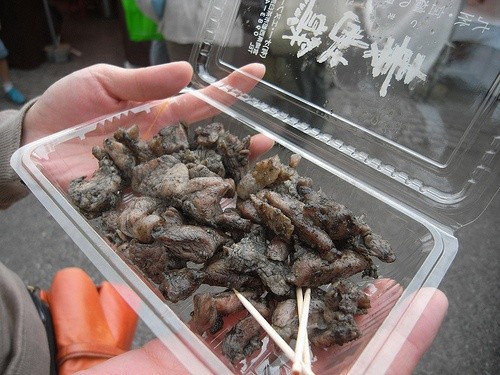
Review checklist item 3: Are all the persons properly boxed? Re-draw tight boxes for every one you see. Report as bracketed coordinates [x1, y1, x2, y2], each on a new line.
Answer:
[237, 0, 330, 129]
[154, 0, 245, 87]
[119, 0, 167, 70]
[0, 59, 449, 375]
